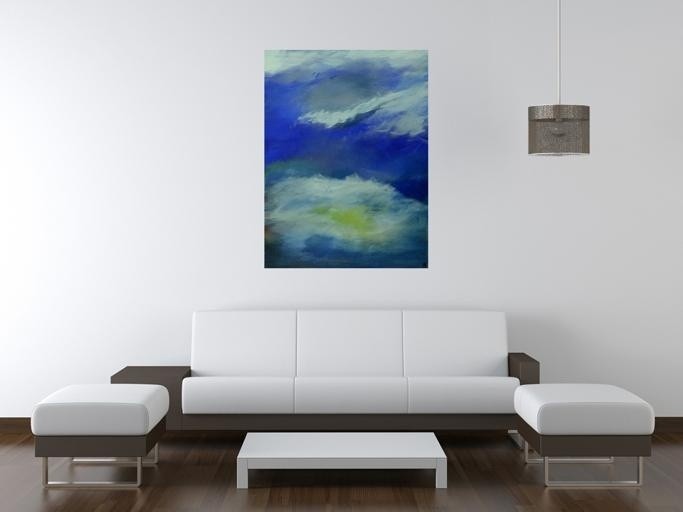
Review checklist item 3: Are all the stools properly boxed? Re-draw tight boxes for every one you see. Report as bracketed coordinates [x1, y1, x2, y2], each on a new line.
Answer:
[30, 383, 170, 490]
[512, 383, 656, 487]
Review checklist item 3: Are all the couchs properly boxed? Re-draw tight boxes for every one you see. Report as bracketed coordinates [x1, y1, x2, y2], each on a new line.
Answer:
[110, 308, 540, 433]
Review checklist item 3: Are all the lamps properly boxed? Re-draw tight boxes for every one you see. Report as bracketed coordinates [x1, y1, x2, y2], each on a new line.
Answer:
[528, 0, 591, 158]
